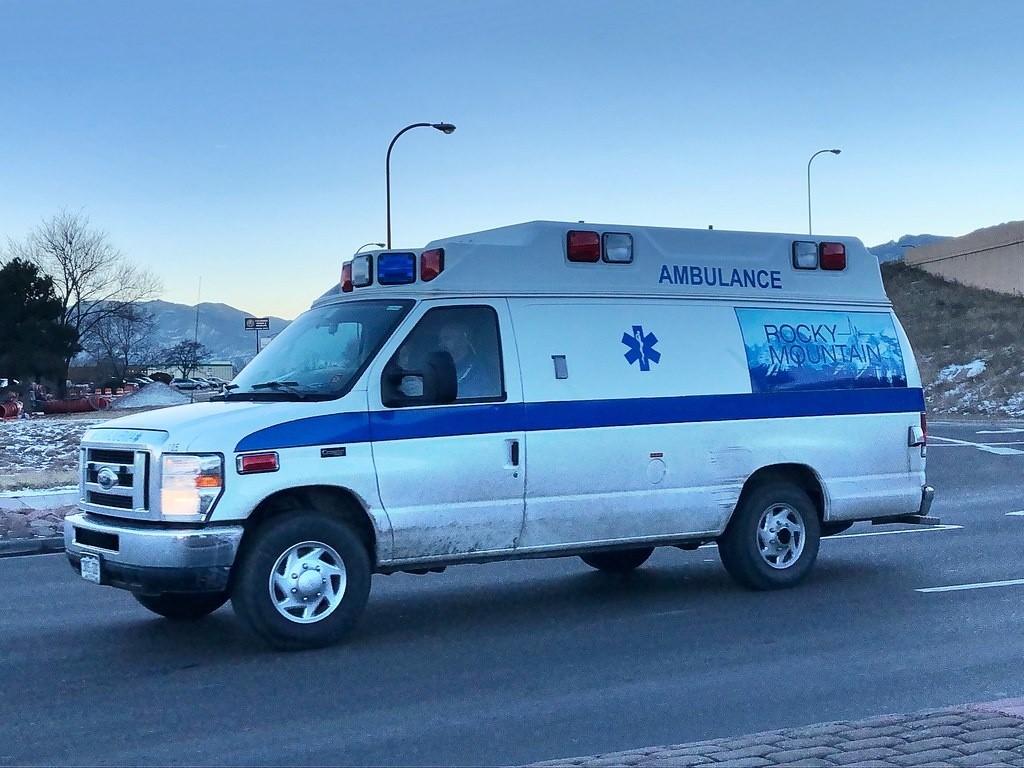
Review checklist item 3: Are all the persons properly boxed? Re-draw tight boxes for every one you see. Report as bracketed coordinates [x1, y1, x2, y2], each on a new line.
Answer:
[398, 341, 424, 371]
[437, 315, 500, 398]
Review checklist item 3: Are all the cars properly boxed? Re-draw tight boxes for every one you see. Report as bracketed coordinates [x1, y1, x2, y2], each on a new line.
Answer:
[208, 376, 230, 387]
[108, 373, 155, 391]
[0, 378, 19, 388]
[169, 377, 210, 390]
[191, 377, 220, 389]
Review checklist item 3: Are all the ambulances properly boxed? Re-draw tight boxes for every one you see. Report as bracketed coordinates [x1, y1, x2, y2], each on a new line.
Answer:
[64, 219, 944, 652]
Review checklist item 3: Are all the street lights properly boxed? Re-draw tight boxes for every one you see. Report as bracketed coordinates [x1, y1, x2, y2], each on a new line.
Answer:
[807, 147, 842, 234]
[385, 123, 459, 250]
